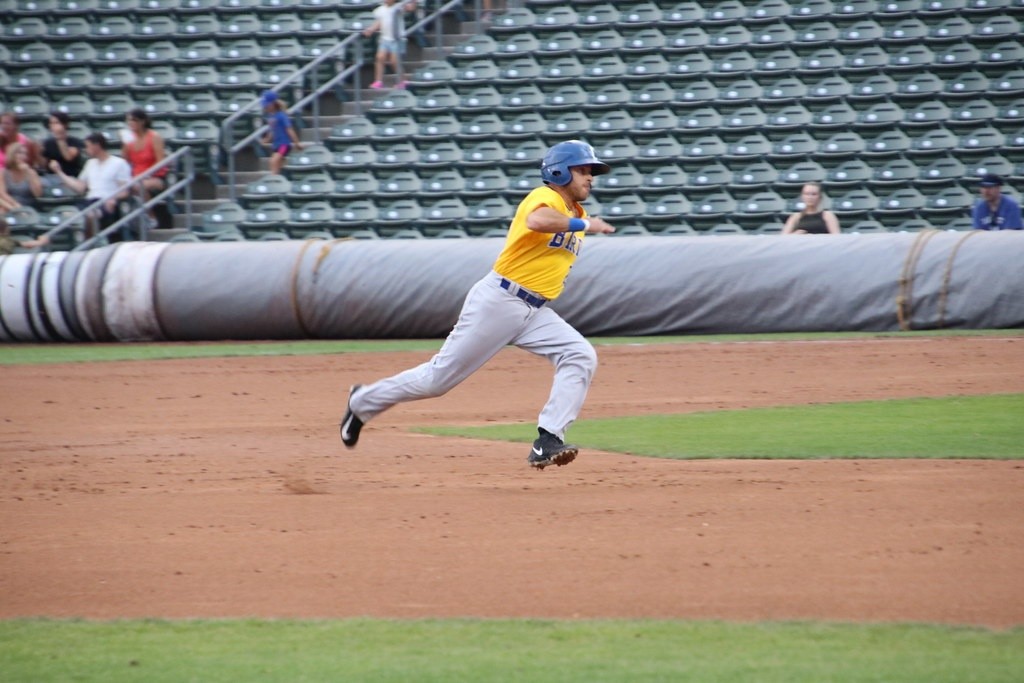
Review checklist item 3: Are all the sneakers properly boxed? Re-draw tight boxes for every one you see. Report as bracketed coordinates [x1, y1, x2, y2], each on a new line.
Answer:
[528, 438, 579, 470]
[339, 384, 362, 449]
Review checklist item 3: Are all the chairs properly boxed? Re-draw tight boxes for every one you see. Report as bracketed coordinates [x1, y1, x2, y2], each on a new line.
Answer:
[0, 0, 1024, 244]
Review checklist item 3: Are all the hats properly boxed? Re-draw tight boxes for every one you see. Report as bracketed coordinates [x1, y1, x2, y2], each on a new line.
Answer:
[260, 91, 276, 107]
[981, 173, 1003, 187]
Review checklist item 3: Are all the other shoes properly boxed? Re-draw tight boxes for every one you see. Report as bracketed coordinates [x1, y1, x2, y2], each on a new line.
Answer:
[371, 81, 382, 89]
[395, 82, 407, 88]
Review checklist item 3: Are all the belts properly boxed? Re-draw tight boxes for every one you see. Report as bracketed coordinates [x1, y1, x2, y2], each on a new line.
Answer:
[502, 278, 546, 308]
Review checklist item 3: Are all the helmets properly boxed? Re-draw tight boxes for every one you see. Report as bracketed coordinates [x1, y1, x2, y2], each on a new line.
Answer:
[542, 140, 611, 187]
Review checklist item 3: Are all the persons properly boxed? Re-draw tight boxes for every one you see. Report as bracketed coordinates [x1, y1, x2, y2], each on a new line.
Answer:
[782, 182, 841, 234]
[121, 109, 169, 229]
[39, 112, 83, 177]
[363, 0, 417, 88]
[49, 133, 131, 239]
[481, 0, 492, 21]
[0, 110, 32, 169]
[973, 173, 1023, 231]
[259, 90, 304, 174]
[0, 142, 42, 236]
[340, 140, 616, 469]
